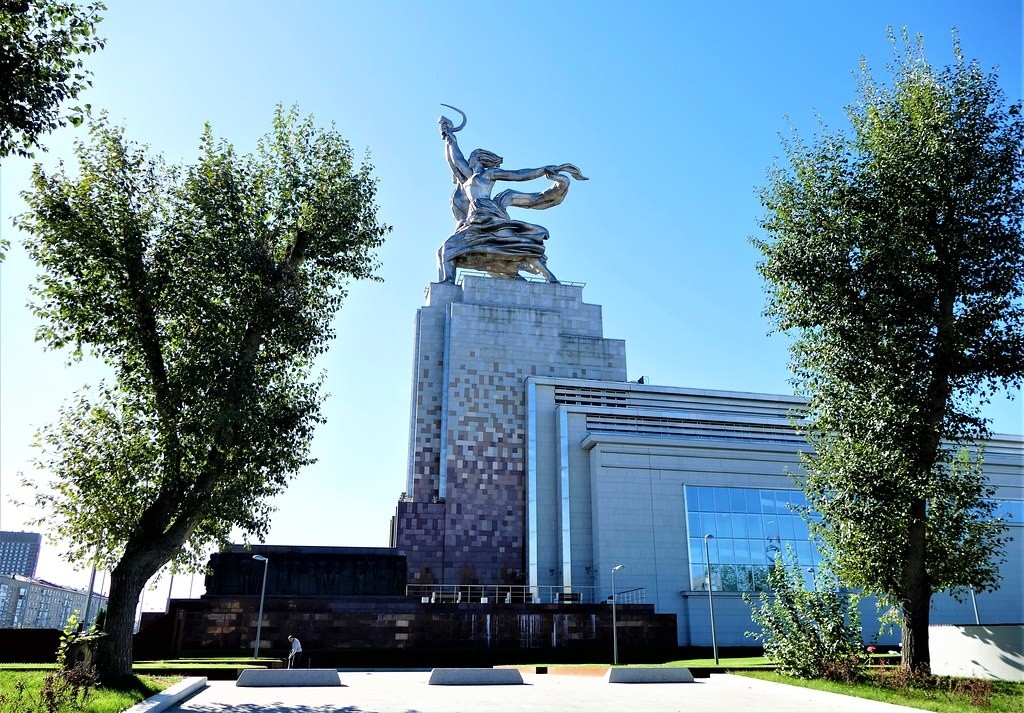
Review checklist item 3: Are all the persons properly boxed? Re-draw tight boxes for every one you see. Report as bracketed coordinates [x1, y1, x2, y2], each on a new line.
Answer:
[288, 635, 302, 669]
[438, 115, 570, 285]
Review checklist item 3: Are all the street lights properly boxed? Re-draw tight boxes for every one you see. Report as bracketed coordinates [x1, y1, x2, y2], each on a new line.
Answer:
[253, 554, 270, 659]
[704, 533, 720, 666]
[611, 564, 625, 664]
[807, 567, 817, 592]
